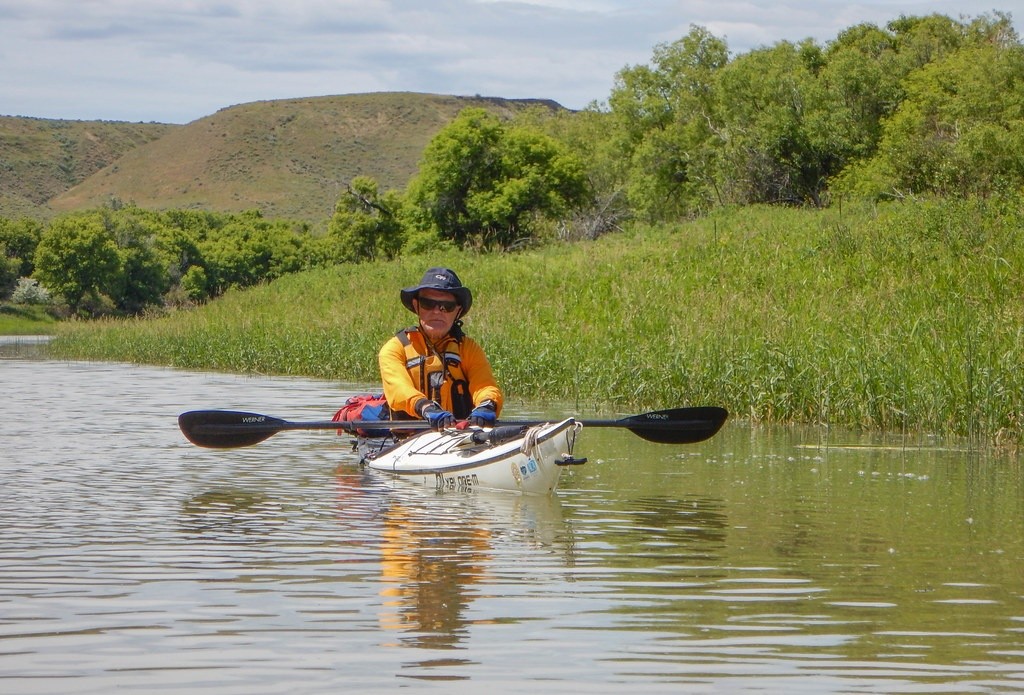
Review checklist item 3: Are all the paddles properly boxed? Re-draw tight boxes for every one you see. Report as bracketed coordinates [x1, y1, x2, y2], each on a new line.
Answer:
[178, 406, 732, 450]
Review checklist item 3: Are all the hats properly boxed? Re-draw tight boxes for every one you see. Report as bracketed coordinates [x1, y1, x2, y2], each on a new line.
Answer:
[400, 268, 472, 320]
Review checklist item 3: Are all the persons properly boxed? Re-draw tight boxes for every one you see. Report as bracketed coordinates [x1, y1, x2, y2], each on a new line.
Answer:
[379, 267, 502, 444]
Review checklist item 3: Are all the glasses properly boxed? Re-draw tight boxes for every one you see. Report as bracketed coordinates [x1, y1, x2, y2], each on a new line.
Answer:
[417, 297, 460, 313]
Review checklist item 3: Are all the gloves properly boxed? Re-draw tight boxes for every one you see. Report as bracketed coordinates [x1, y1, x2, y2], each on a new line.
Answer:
[422, 400, 456, 433]
[467, 399, 497, 428]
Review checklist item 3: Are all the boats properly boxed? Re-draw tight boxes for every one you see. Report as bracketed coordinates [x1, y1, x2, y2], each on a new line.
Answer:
[339, 393, 577, 495]
